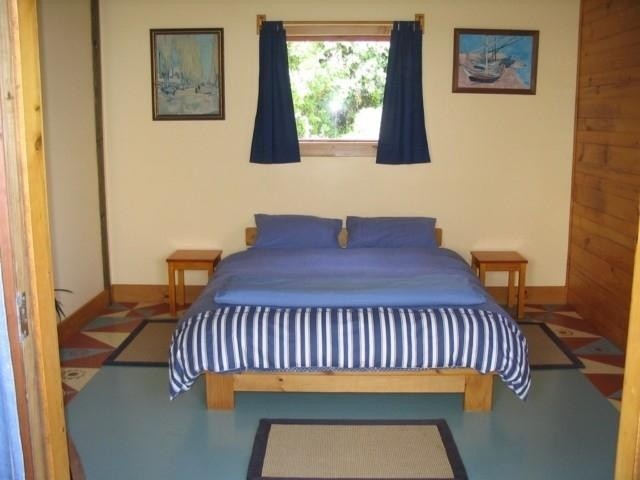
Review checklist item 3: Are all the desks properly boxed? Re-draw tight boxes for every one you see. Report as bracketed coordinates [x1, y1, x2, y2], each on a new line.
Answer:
[471, 251, 528, 319]
[165, 248, 223, 318]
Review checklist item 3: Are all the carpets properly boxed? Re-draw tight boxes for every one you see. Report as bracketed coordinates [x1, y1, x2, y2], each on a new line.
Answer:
[100, 317, 177, 367]
[517, 321, 586, 371]
[244, 417, 471, 480]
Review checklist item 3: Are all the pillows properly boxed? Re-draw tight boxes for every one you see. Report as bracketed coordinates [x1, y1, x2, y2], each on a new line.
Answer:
[250, 213, 439, 249]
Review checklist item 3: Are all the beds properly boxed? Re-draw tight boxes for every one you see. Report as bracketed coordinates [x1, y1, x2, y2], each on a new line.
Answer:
[204, 226, 497, 416]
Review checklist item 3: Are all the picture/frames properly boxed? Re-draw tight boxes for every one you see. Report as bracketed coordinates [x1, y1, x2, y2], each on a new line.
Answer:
[452, 26, 537, 96]
[150, 27, 226, 121]
